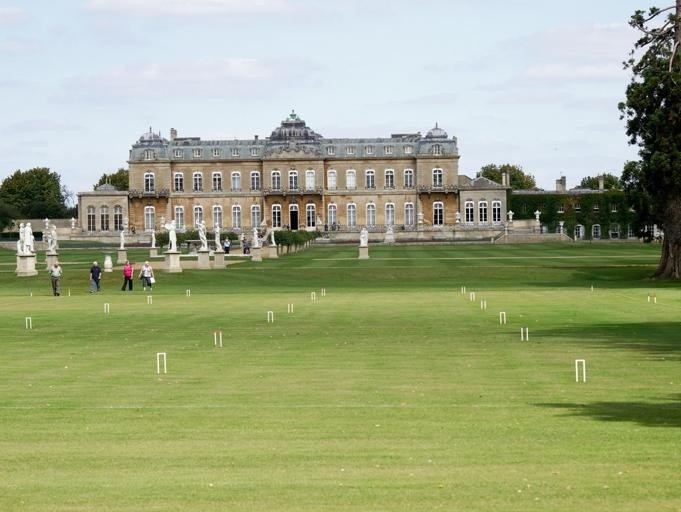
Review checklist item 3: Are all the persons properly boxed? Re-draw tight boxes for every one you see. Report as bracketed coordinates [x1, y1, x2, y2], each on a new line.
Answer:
[224, 238, 231, 255]
[211, 223, 225, 251]
[169, 220, 178, 252]
[89, 259, 101, 294]
[151, 231, 156, 247]
[120, 260, 133, 290]
[359, 226, 370, 246]
[22, 223, 34, 251]
[196, 218, 209, 250]
[19, 221, 38, 252]
[120, 230, 126, 249]
[49, 262, 65, 296]
[139, 261, 155, 292]
[50, 225, 56, 251]
[242, 236, 251, 254]
